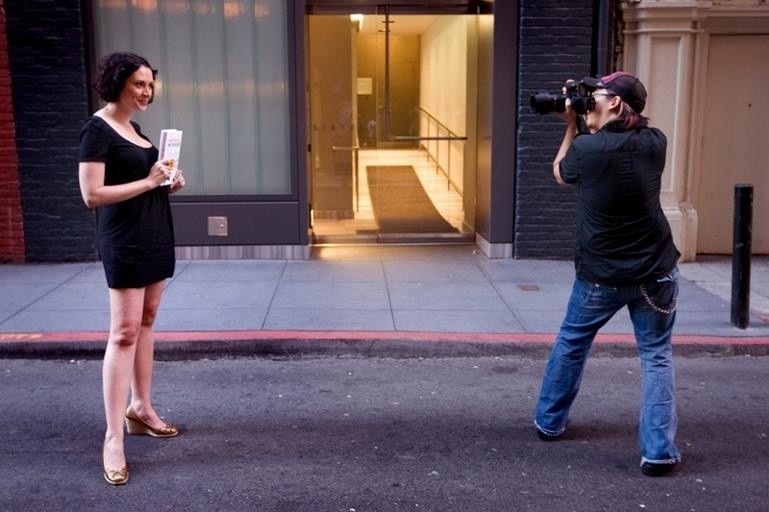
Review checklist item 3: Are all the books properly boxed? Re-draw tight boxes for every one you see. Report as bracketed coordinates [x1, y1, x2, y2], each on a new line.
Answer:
[157, 127, 182, 187]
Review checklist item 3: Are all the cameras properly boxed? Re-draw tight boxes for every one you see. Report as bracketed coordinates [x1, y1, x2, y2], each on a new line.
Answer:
[528, 78, 596, 117]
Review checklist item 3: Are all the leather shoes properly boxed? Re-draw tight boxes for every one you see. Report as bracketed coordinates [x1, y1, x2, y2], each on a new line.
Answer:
[103, 439, 129, 485]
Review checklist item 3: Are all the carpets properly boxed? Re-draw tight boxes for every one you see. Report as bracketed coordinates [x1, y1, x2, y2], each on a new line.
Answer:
[356, 165, 460, 235]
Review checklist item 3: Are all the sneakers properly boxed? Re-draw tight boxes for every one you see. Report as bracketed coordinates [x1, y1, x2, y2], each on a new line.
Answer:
[641, 455, 680, 476]
[535, 418, 564, 441]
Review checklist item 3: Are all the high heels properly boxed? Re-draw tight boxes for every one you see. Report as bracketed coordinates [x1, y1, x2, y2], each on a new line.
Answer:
[125, 405, 179, 438]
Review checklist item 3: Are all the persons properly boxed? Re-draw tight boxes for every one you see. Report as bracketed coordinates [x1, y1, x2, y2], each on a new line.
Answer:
[75, 50, 188, 485]
[532, 69, 684, 479]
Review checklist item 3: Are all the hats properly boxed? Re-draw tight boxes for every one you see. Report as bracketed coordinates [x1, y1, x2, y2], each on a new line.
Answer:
[584, 70, 648, 115]
